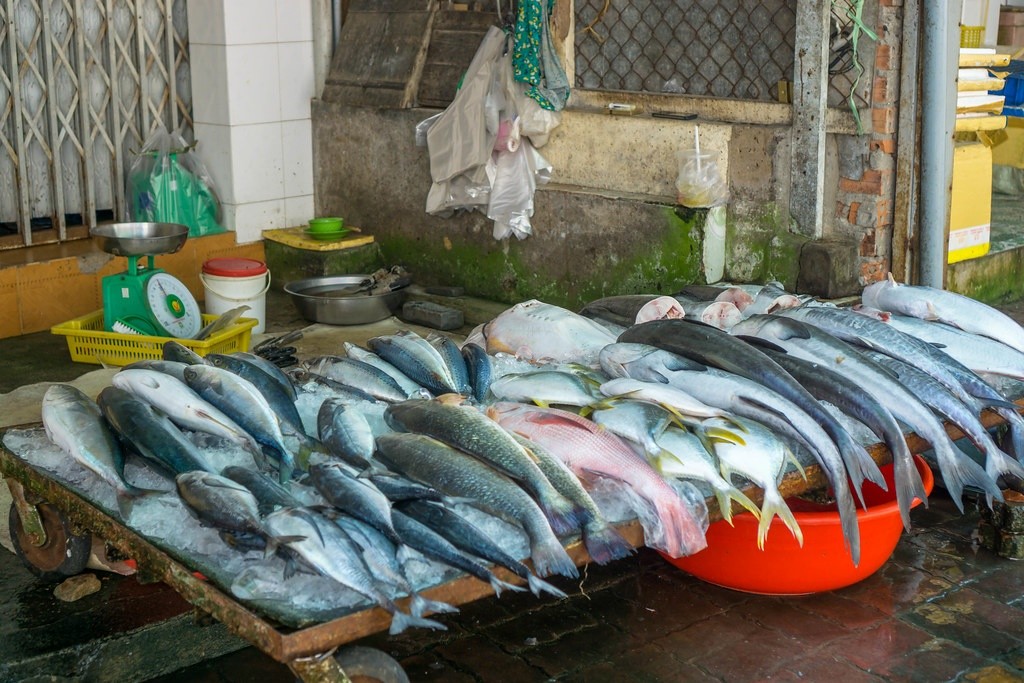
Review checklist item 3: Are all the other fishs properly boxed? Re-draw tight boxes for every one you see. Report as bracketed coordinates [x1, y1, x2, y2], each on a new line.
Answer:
[40, 272, 1023, 637]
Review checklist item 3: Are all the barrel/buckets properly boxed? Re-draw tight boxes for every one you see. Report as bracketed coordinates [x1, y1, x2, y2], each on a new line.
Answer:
[200, 255, 272, 337]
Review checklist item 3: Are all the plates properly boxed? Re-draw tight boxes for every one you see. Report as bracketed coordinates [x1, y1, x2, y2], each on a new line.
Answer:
[304, 226, 350, 240]
[89, 219, 190, 258]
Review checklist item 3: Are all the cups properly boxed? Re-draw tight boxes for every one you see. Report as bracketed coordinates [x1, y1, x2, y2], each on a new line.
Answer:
[673, 146, 719, 210]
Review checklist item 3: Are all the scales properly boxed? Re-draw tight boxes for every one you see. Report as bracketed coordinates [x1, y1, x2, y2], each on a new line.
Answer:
[125, 128, 227, 239]
[87, 221, 203, 351]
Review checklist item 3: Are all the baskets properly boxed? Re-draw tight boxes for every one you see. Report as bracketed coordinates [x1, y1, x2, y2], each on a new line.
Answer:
[52, 309, 256, 366]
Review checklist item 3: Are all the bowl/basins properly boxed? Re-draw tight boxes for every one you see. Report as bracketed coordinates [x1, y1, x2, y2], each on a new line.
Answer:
[308, 216, 344, 232]
[284, 273, 405, 326]
[651, 450, 939, 598]
